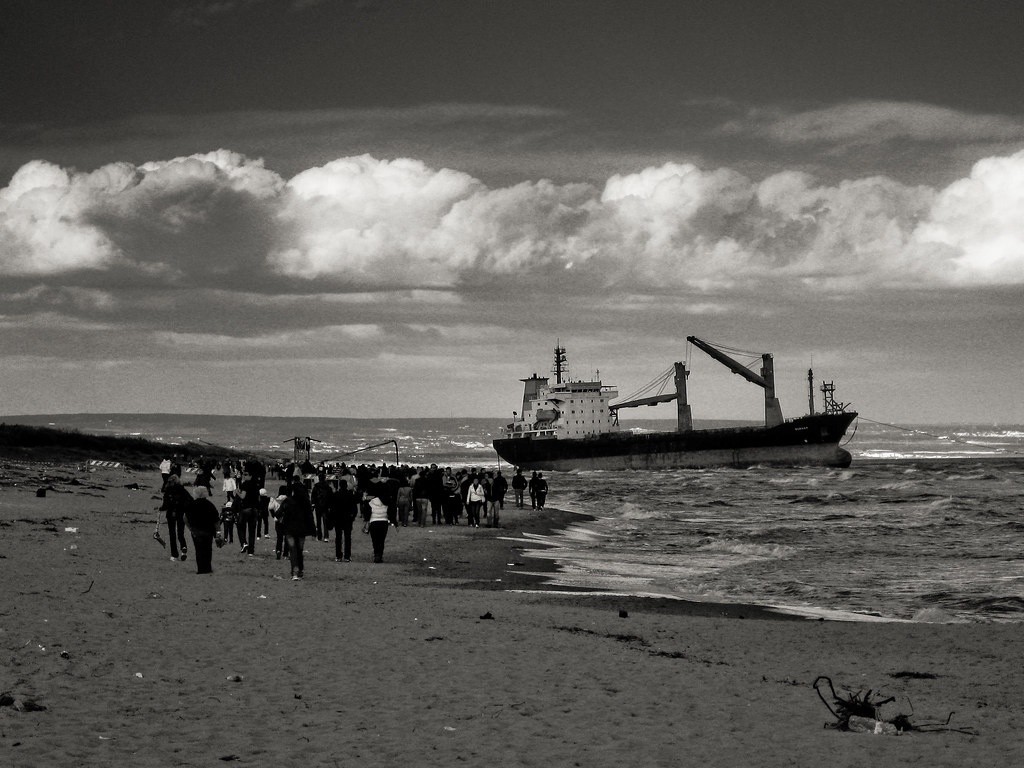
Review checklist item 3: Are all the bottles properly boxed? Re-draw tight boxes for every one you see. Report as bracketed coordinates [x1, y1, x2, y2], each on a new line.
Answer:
[848, 714, 903, 736]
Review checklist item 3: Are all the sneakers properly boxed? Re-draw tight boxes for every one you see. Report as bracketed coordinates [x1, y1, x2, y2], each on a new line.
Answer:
[256, 537, 260, 541]
[265, 535, 270, 538]
[249, 553, 253, 557]
[239, 543, 249, 553]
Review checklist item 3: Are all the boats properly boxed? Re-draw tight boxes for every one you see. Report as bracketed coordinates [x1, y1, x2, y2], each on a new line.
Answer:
[493, 335, 858, 472]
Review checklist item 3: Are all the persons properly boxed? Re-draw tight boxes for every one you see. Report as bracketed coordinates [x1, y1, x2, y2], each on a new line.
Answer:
[158, 454, 548, 580]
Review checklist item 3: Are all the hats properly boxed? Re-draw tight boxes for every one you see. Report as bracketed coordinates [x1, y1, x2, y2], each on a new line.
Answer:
[226, 501, 232, 507]
[193, 486, 208, 498]
[260, 489, 266, 495]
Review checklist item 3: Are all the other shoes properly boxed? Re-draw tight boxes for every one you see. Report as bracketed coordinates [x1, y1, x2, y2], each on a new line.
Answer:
[475, 524, 479, 528]
[170, 557, 177, 561]
[335, 557, 353, 563]
[292, 575, 304, 581]
[276, 550, 290, 560]
[449, 523, 458, 526]
[316, 536, 329, 542]
[180, 547, 187, 561]
